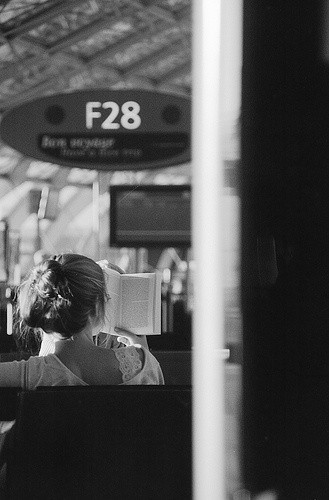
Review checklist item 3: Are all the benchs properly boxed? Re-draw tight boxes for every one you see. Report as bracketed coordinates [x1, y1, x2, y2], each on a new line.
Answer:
[1, 385, 253, 500]
[1, 351, 192, 386]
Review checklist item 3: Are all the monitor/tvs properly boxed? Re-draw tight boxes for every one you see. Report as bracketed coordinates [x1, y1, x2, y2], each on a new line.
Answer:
[110, 184, 191, 248]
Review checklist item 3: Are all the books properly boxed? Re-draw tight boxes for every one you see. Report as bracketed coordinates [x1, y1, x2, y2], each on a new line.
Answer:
[98, 265, 161, 335]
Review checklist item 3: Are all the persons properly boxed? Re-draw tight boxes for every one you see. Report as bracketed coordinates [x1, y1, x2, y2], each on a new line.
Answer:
[0, 253, 165, 385]
